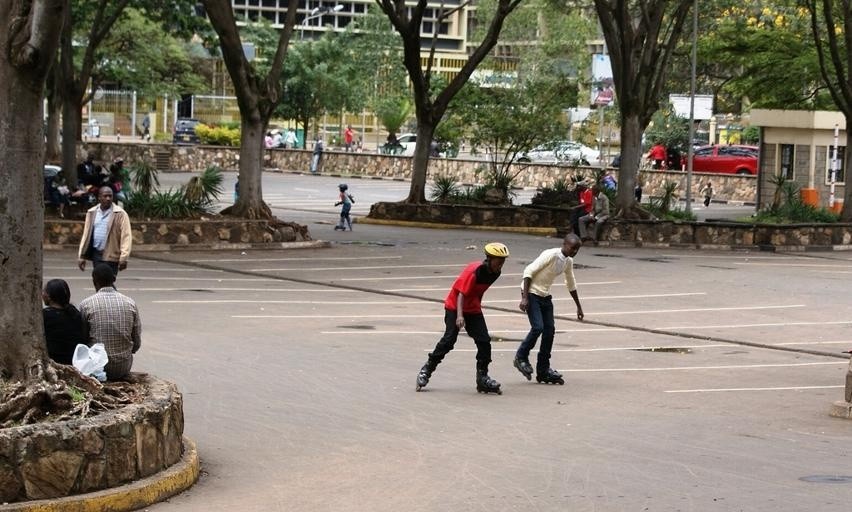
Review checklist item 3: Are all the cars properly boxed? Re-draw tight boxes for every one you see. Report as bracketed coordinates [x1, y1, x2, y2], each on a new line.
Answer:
[679, 141, 759, 174]
[374, 132, 453, 159]
[58, 117, 101, 138]
[43, 165, 98, 209]
[509, 137, 603, 166]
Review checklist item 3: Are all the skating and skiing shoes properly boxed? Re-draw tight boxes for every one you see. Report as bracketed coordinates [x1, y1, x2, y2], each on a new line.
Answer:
[513, 351, 533, 382]
[416, 360, 432, 391]
[475, 368, 502, 396]
[536, 363, 564, 385]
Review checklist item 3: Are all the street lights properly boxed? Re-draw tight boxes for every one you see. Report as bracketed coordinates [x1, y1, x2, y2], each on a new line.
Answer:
[293, 3, 344, 150]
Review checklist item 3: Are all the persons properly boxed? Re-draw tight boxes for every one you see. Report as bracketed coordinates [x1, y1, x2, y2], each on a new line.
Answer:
[333, 184, 354, 232]
[701, 182, 713, 207]
[142, 112, 151, 133]
[79, 264, 141, 380]
[78, 186, 132, 276]
[646, 142, 688, 172]
[593, 84, 615, 106]
[264, 127, 299, 148]
[234, 173, 240, 194]
[415, 243, 510, 395]
[344, 124, 355, 152]
[573, 155, 622, 247]
[513, 233, 584, 385]
[309, 136, 322, 172]
[430, 137, 440, 157]
[42, 113, 131, 218]
[42, 279, 90, 365]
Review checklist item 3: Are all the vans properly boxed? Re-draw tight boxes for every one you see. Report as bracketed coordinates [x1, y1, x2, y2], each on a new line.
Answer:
[171, 117, 207, 145]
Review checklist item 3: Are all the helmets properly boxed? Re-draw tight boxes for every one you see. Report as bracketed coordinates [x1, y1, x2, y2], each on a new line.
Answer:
[483, 242, 510, 258]
[338, 183, 350, 189]
